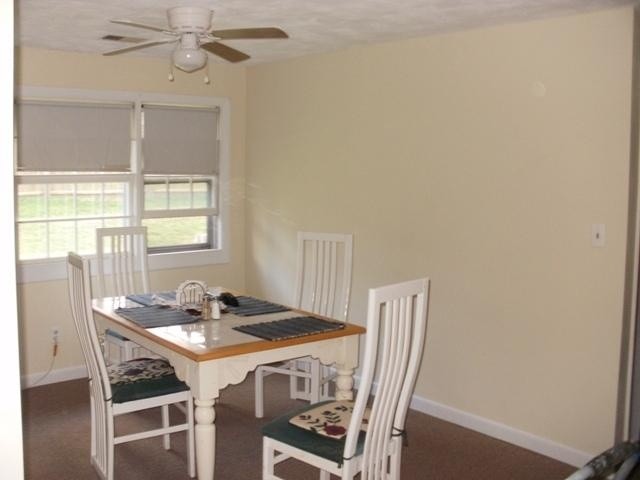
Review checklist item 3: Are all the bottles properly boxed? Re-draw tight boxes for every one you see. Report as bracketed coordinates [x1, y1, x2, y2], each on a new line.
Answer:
[201, 295, 221, 321]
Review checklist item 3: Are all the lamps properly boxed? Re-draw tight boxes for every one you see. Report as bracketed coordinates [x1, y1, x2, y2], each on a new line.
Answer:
[165, 41, 212, 84]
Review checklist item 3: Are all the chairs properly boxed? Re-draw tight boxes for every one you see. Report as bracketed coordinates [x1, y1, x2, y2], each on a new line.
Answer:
[65, 224, 200, 479]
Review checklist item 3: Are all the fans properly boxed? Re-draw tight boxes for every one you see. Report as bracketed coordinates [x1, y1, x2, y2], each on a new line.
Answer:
[97, 2, 293, 63]
